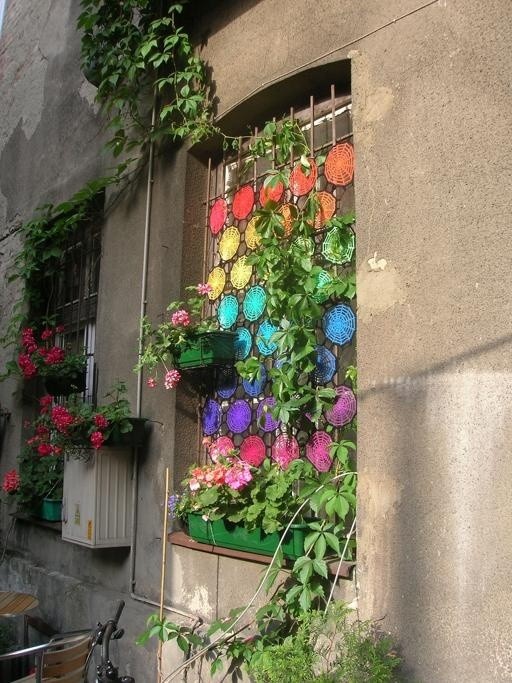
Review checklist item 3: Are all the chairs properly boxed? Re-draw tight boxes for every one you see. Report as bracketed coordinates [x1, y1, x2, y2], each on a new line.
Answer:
[0, 629, 92, 683]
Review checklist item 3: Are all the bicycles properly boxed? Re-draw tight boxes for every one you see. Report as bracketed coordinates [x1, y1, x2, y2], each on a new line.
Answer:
[81, 593, 142, 682]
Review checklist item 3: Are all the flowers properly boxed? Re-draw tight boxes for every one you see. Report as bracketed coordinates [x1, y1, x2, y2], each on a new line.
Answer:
[0, 467, 63, 504]
[167, 438, 312, 533]
[18, 327, 88, 388]
[132, 283, 213, 392]
[23, 381, 133, 464]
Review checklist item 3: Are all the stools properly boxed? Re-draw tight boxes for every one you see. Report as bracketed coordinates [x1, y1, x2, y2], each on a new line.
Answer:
[0, 592, 39, 676]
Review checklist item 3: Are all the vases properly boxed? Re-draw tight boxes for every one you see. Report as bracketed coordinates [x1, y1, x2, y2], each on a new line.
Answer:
[87, 418, 148, 446]
[44, 371, 87, 394]
[29, 497, 63, 522]
[186, 508, 325, 561]
[167, 329, 235, 371]
